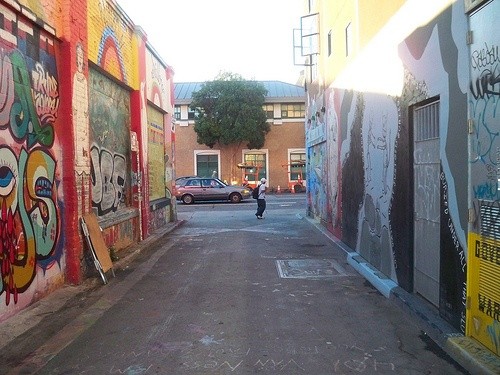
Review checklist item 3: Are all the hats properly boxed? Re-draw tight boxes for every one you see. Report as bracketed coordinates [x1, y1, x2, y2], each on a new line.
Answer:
[261, 177, 267, 183]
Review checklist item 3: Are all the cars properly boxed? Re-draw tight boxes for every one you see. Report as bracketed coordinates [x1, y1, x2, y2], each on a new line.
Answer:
[174, 176, 253, 205]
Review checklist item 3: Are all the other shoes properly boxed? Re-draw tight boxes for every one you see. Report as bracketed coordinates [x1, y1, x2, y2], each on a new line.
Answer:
[257, 216, 265, 219]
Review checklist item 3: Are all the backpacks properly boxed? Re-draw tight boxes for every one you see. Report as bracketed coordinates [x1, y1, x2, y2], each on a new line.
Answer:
[252, 183, 262, 199]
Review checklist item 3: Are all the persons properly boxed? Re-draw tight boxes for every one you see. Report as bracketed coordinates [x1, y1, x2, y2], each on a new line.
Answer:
[255, 178, 268, 219]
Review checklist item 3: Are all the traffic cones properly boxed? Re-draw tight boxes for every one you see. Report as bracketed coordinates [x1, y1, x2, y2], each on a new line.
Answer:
[291, 185, 295, 194]
[277, 184, 281, 194]
